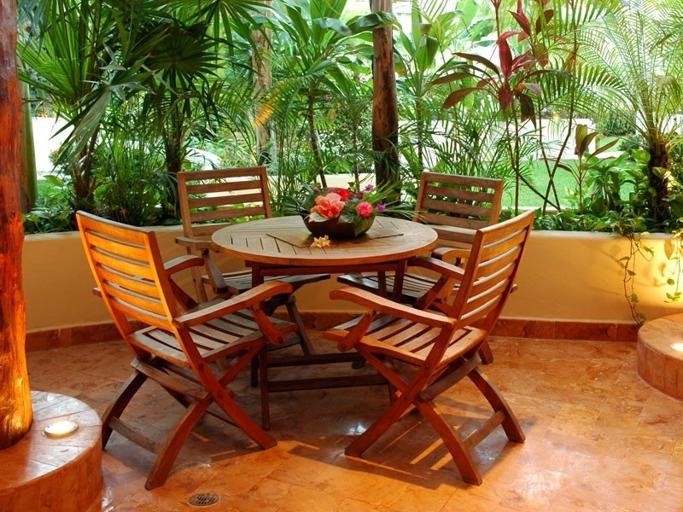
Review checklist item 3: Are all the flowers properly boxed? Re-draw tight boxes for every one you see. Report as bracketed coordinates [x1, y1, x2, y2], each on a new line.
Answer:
[300, 181, 383, 225]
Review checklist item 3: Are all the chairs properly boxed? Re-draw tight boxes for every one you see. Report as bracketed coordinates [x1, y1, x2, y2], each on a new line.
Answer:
[337, 169, 506, 370]
[75, 209, 296, 490]
[321, 207, 539, 486]
[173, 167, 332, 357]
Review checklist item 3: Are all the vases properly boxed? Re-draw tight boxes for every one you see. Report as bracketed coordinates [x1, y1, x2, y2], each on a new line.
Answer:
[302, 212, 378, 241]
